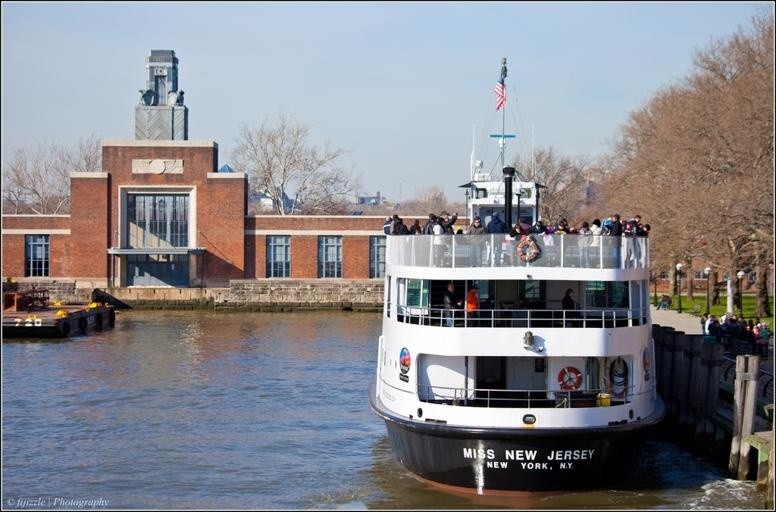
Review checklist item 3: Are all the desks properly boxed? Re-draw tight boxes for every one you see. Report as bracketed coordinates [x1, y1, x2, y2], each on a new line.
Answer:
[4, 290, 50, 313]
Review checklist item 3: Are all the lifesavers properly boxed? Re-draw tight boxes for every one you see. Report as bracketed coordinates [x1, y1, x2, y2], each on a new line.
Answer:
[516, 239, 540, 262]
[558, 367, 583, 389]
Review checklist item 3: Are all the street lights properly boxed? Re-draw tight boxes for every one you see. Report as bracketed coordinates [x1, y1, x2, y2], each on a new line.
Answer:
[676, 263, 683, 313]
[704, 267, 711, 314]
[736, 271, 745, 318]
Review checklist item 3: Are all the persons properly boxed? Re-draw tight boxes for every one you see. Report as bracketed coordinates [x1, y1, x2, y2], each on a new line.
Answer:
[562, 288, 581, 327]
[443, 283, 479, 327]
[700, 312, 772, 360]
[383, 210, 650, 267]
[656, 294, 672, 310]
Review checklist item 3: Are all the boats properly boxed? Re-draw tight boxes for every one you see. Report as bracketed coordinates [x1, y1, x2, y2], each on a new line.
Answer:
[2, 278, 115, 336]
[368, 58, 667, 491]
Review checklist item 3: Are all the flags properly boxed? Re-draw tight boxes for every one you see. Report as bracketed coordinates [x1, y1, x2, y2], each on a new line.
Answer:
[494, 58, 508, 111]
